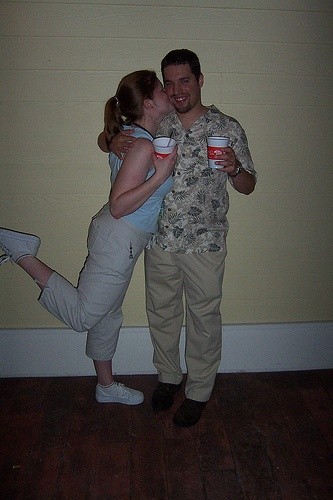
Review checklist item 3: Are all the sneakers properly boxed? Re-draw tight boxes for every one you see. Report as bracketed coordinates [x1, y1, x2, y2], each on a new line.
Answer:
[0, 228, 41, 265]
[95, 383, 144, 405]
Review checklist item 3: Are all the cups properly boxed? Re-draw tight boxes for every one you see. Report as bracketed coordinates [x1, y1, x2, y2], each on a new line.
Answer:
[208, 136, 229, 169]
[153, 137, 176, 159]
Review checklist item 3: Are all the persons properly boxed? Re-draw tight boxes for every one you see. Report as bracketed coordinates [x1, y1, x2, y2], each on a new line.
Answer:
[1, 70, 179, 405]
[97, 48, 258, 429]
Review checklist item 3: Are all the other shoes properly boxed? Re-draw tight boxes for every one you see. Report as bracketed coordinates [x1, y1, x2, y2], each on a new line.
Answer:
[173, 398, 207, 427]
[151, 382, 182, 411]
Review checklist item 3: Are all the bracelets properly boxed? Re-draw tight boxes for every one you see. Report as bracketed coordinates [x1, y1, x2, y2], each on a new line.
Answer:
[105, 131, 119, 151]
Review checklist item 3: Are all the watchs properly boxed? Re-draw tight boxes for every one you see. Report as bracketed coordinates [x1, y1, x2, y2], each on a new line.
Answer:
[229, 162, 242, 178]
